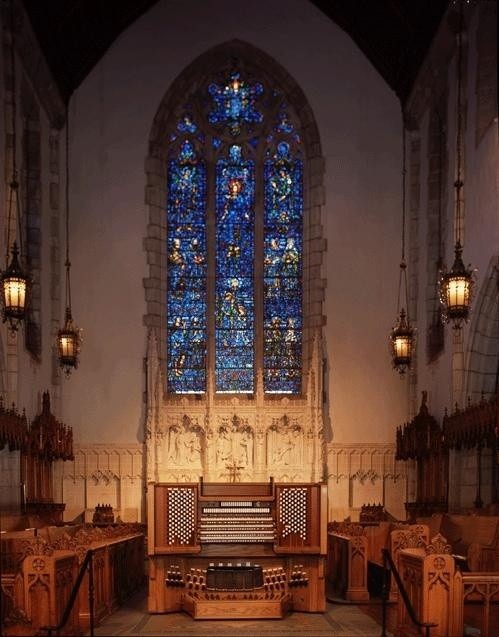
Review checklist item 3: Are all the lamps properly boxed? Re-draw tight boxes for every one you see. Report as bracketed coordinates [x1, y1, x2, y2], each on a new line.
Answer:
[60, 86, 83, 375]
[437, 0, 475, 329]
[0, 0, 33, 333]
[387, 88, 415, 375]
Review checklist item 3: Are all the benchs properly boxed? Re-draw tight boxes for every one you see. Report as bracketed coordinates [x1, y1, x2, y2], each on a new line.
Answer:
[0, 521, 147, 637]
[327, 508, 499, 637]
